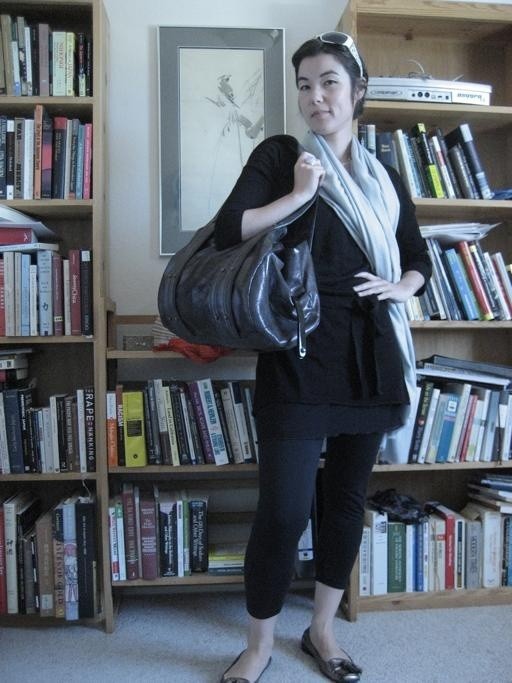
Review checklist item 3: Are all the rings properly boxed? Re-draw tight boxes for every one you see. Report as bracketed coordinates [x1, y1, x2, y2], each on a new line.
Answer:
[306, 156, 317, 165]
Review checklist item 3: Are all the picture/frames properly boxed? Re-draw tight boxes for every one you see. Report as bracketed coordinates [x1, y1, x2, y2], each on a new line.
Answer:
[155, 25, 293, 255]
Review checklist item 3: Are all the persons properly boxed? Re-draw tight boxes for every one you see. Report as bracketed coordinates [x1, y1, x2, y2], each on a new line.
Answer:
[209, 29, 434, 683]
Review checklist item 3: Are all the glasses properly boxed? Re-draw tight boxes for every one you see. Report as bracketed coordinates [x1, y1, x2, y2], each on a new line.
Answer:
[311, 31, 364, 78]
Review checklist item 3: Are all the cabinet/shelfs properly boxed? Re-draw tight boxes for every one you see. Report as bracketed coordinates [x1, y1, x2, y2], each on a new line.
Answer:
[107, 311, 344, 595]
[333, 2, 512, 623]
[0, 1, 117, 635]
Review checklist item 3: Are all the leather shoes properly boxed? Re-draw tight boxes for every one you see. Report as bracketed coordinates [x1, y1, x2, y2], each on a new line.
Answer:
[300, 625, 362, 682]
[221, 647, 272, 683]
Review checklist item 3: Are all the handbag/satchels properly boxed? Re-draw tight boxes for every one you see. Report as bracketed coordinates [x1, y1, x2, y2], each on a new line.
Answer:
[157, 143, 322, 360]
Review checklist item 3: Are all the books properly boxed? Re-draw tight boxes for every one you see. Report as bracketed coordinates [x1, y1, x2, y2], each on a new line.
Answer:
[0, 347, 98, 475]
[1, 14, 94, 96]
[402, 222, 512, 322]
[105, 372, 260, 585]
[354, 123, 494, 200]
[374, 352, 510, 465]
[1, 205, 93, 336]
[0, 487, 105, 623]
[0, 105, 93, 200]
[358, 473, 511, 597]
